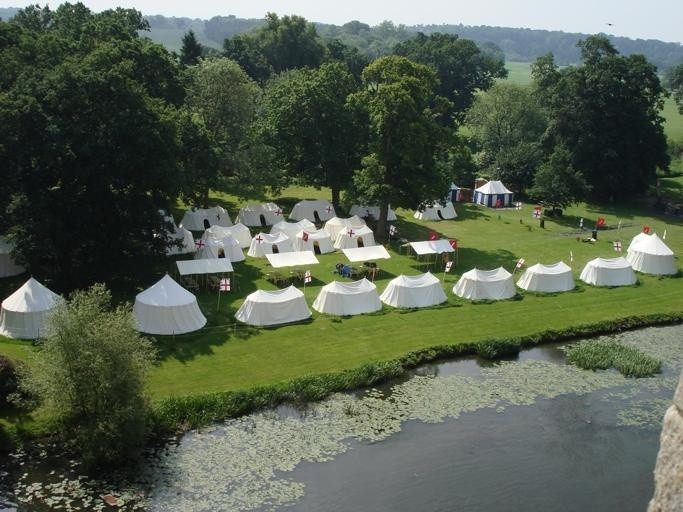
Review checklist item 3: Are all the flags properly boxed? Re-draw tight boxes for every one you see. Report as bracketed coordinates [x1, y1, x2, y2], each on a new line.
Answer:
[612, 241, 622, 252]
[515, 202, 522, 210]
[643, 227, 650, 233]
[304, 269, 312, 283]
[445, 261, 452, 272]
[596, 217, 604, 225]
[516, 258, 524, 268]
[532, 210, 541, 218]
[219, 278, 231, 292]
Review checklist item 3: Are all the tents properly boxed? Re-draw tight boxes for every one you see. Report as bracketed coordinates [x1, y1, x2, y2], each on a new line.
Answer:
[234, 285, 313, 327]
[626, 231, 651, 253]
[0, 277, 69, 340]
[312, 277, 382, 316]
[626, 232, 679, 275]
[130, 273, 207, 336]
[515, 261, 576, 293]
[578, 256, 637, 287]
[452, 267, 517, 301]
[378, 272, 449, 309]
